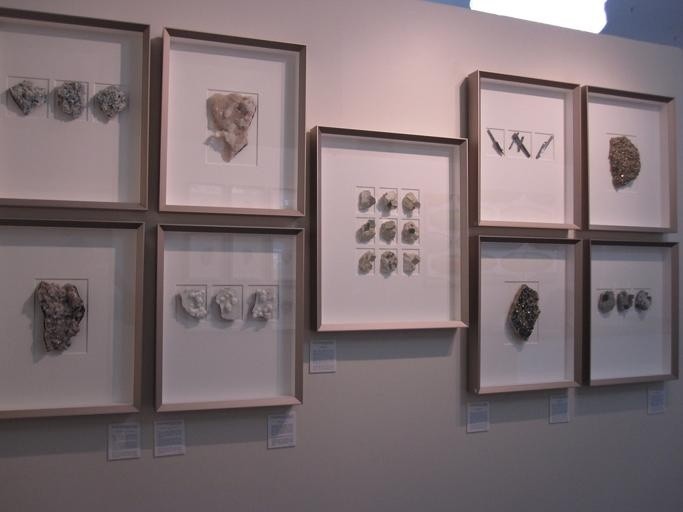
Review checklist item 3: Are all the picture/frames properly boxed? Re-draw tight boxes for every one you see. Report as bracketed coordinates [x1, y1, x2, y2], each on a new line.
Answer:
[582, 85, 678, 233]
[0, 218, 146, 419]
[309, 125, 468, 333]
[468, 70, 582, 230]
[583, 238, 681, 387]
[1, 5, 151, 212]
[468, 236, 583, 395]
[156, 223, 307, 412]
[158, 27, 306, 217]
